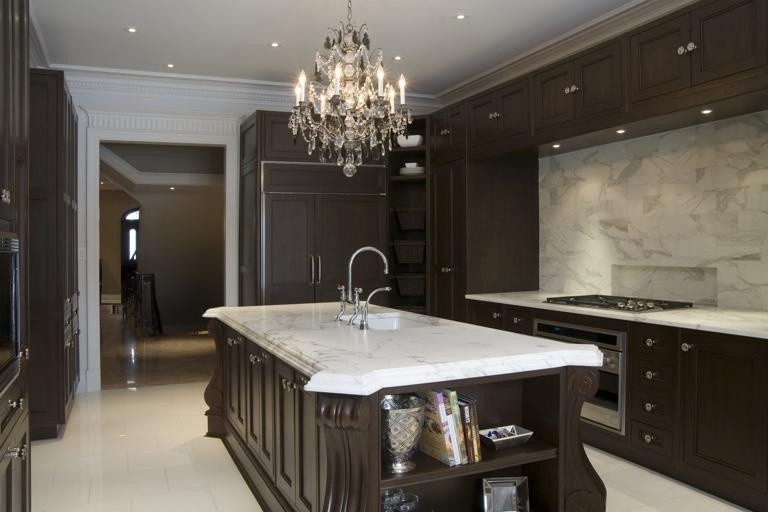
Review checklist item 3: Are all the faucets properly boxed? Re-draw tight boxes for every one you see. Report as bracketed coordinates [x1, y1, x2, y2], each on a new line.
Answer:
[348, 246, 389, 301]
[364, 286, 392, 322]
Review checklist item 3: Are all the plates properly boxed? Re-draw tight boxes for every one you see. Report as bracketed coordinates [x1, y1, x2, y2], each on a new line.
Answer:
[399, 167, 425, 176]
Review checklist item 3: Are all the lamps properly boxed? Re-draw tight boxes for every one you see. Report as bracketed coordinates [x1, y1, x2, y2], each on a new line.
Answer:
[287, 0, 413, 178]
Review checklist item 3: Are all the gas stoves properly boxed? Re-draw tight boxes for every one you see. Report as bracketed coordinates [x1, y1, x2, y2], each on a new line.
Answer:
[546, 294, 694, 314]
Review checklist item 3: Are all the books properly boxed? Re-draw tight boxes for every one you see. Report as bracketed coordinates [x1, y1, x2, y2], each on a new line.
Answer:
[420, 388, 482, 467]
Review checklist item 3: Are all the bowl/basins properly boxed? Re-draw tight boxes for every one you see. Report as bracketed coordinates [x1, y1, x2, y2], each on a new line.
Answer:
[397, 135, 423, 147]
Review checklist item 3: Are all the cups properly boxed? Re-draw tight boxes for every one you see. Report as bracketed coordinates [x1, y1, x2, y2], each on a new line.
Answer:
[405, 162, 417, 167]
[384, 488, 419, 512]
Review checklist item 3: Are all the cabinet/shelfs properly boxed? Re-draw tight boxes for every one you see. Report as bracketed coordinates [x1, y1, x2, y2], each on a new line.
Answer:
[0, 0, 33, 512]
[476, 300, 532, 335]
[628, 321, 680, 482]
[629, 1, 768, 127]
[530, 34, 622, 154]
[238, 110, 389, 307]
[274, 362, 319, 512]
[389, 114, 432, 316]
[431, 101, 538, 335]
[377, 372, 563, 512]
[220, 325, 247, 446]
[244, 340, 276, 484]
[680, 327, 768, 512]
[465, 73, 530, 163]
[31, 68, 80, 441]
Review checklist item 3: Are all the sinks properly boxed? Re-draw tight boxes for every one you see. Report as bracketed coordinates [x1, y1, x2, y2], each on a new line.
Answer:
[335, 313, 440, 331]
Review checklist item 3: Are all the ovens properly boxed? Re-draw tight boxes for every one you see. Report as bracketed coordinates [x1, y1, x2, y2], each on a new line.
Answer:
[532, 318, 626, 437]
